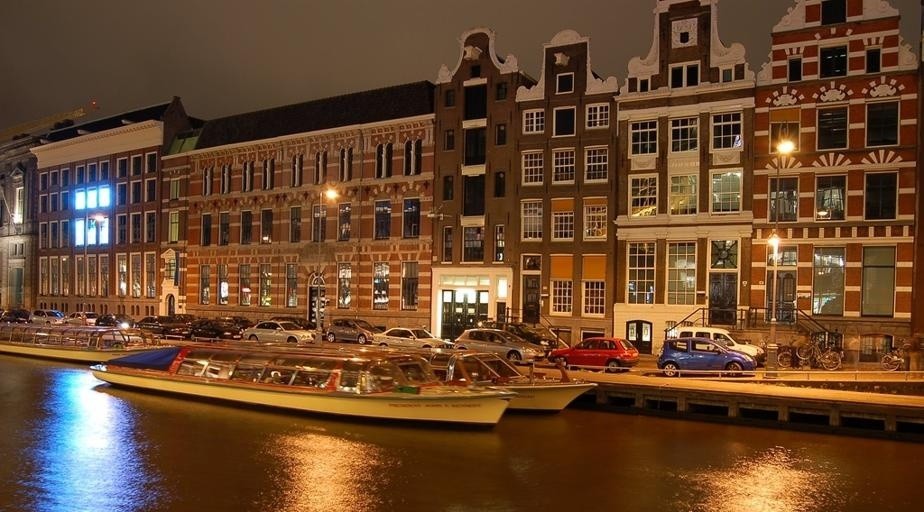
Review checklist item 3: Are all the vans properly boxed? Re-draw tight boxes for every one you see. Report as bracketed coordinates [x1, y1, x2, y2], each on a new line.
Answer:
[676, 326, 766, 363]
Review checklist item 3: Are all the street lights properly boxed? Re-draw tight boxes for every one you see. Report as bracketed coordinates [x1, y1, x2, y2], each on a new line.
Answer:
[6, 213, 23, 317]
[313, 190, 337, 350]
[764, 140, 795, 377]
[81, 215, 104, 325]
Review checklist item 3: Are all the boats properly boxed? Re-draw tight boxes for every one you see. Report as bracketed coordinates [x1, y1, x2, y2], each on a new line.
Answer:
[401, 348, 600, 416]
[89, 349, 519, 431]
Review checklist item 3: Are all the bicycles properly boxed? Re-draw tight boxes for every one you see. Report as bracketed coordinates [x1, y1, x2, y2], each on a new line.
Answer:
[881, 346, 904, 371]
[777, 332, 842, 371]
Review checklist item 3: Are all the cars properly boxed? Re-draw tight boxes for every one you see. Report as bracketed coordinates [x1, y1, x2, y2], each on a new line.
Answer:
[371, 327, 455, 349]
[28, 309, 65, 326]
[547, 337, 639, 373]
[657, 337, 757, 377]
[94, 313, 136, 327]
[62, 311, 99, 326]
[1, 309, 33, 324]
[135, 314, 326, 343]
[326, 319, 383, 345]
[453, 321, 556, 366]
